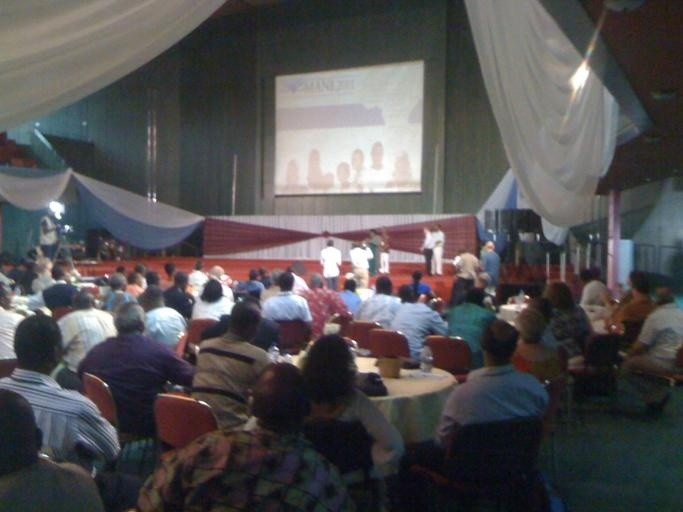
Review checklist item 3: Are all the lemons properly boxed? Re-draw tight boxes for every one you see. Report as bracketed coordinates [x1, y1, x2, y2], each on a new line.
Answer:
[374, 355, 405, 378]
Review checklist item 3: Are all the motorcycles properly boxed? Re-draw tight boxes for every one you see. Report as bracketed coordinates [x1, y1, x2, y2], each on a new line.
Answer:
[51, 234, 82, 278]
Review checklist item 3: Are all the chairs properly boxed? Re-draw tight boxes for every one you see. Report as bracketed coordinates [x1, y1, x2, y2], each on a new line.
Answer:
[1, 284, 681, 511]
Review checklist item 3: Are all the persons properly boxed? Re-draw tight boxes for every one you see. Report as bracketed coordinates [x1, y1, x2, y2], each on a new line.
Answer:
[421, 222, 445, 275]
[479, 240, 500, 286]
[285, 143, 412, 193]
[39, 208, 61, 261]
[365, 228, 391, 273]
[320, 240, 342, 290]
[0, 255, 683, 512]
[350, 242, 373, 288]
[449, 244, 480, 308]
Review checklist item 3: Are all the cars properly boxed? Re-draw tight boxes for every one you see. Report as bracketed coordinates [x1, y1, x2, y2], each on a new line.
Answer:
[417, 356, 432, 372]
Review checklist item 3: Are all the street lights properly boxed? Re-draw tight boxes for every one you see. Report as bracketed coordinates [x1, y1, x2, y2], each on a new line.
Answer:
[356, 371, 389, 396]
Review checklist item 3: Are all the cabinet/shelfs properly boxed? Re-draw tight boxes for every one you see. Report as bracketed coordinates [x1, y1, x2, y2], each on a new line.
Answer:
[282, 351, 292, 364]
[268, 342, 279, 361]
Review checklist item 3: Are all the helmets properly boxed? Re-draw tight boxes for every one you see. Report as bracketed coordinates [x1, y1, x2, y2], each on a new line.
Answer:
[374, 358, 418, 369]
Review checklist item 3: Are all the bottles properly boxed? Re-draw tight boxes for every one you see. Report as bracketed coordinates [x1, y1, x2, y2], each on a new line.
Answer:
[235, 280, 265, 296]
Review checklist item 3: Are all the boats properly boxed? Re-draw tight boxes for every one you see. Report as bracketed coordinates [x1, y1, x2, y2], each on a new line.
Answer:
[484, 209, 498, 233]
[500, 209, 514, 233]
[513, 209, 542, 233]
